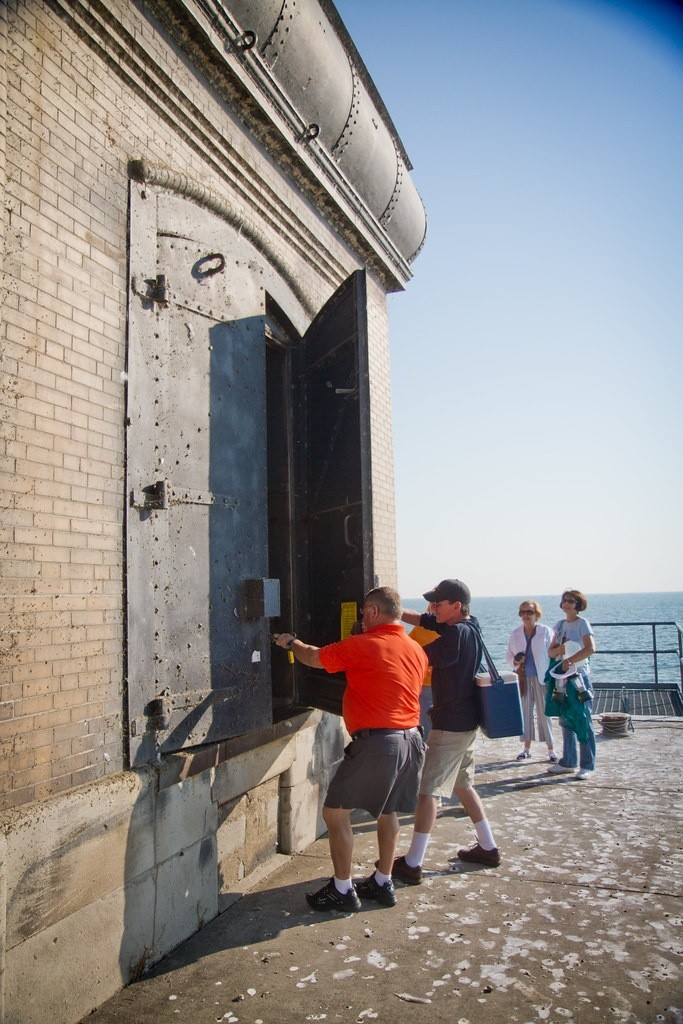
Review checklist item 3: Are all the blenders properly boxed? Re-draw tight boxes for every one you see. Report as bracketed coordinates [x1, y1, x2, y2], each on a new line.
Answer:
[551, 679, 568, 701]
[569, 672, 594, 703]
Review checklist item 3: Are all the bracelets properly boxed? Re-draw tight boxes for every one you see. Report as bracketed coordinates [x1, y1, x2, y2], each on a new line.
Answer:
[286, 638, 298, 651]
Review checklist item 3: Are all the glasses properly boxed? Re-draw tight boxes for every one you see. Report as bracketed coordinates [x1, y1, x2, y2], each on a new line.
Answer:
[562, 599, 580, 605]
[360, 604, 379, 615]
[519, 609, 537, 617]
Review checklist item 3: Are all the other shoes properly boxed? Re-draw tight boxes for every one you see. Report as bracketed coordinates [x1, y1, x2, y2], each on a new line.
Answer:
[575, 768, 595, 780]
[547, 764, 577, 774]
[517, 751, 531, 759]
[550, 754, 559, 764]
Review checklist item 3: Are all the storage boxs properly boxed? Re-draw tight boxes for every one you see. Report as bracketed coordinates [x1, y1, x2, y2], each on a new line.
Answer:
[475, 670, 524, 739]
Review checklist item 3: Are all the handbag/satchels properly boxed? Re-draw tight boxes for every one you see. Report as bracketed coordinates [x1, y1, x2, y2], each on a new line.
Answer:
[513, 663, 526, 697]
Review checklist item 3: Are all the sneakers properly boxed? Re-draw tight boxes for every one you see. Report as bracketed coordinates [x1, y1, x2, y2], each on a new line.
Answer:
[457, 840, 501, 868]
[305, 875, 362, 912]
[354, 870, 397, 907]
[374, 855, 422, 884]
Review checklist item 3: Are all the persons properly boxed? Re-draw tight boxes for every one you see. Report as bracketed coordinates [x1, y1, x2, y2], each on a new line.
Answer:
[542, 589, 596, 780]
[407, 587, 442, 807]
[275, 586, 429, 912]
[506, 600, 559, 764]
[362, 578, 502, 885]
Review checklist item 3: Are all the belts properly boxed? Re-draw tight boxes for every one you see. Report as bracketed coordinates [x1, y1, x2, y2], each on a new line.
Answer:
[351, 726, 419, 741]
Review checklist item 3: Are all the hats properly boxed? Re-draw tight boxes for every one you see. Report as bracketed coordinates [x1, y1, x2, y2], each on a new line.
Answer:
[422, 579, 471, 605]
[549, 660, 577, 680]
